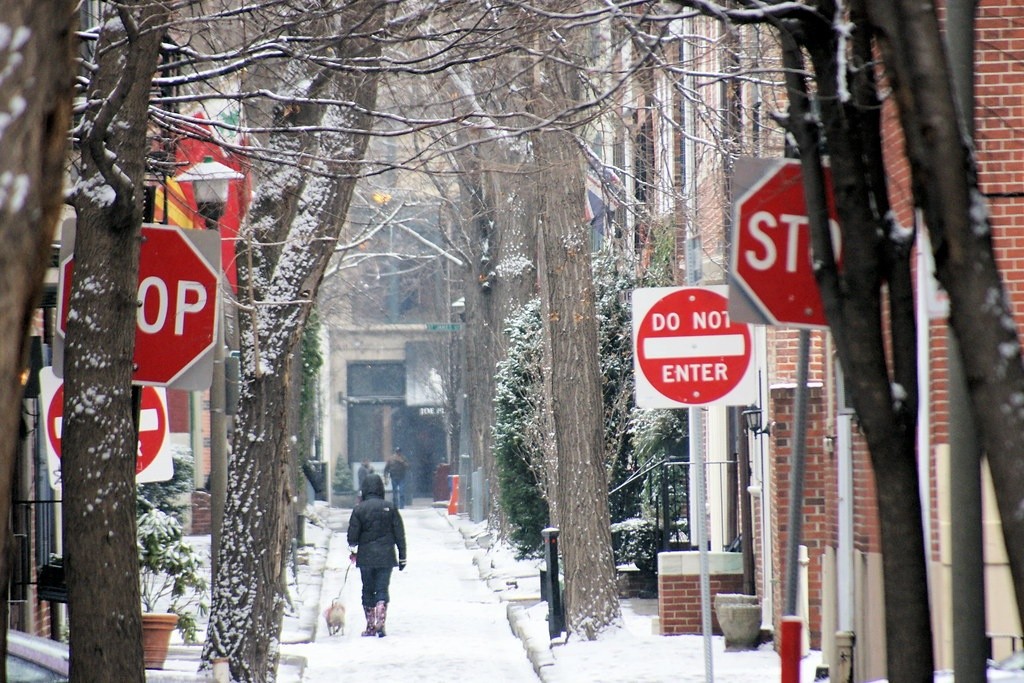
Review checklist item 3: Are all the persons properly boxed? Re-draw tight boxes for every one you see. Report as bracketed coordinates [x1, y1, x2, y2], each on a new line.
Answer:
[384, 444, 411, 511]
[357, 456, 375, 500]
[347, 472, 407, 637]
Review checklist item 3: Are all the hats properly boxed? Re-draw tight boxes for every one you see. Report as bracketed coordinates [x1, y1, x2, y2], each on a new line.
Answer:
[394, 446, 401, 454]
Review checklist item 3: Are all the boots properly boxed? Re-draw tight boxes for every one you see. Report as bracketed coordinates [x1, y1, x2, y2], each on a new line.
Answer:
[361, 607, 376, 636]
[375, 599, 387, 638]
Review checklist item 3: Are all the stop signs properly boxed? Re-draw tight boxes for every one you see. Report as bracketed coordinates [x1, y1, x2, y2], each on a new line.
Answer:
[40, 363, 173, 489]
[633, 285, 767, 410]
[728, 157, 888, 330]
[55, 220, 217, 390]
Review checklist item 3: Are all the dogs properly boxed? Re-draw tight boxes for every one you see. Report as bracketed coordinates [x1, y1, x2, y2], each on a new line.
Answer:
[322, 605, 345, 636]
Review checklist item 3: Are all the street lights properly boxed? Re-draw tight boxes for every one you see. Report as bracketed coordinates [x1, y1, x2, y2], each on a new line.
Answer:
[172, 157, 245, 683]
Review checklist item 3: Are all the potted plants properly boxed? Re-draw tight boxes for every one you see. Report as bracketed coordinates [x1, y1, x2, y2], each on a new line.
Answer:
[136, 509, 209, 670]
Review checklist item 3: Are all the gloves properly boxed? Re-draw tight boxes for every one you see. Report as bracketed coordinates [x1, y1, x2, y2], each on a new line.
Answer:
[398, 559, 407, 571]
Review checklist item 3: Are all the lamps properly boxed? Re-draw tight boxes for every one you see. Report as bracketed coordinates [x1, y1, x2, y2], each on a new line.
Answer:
[740, 404, 770, 440]
